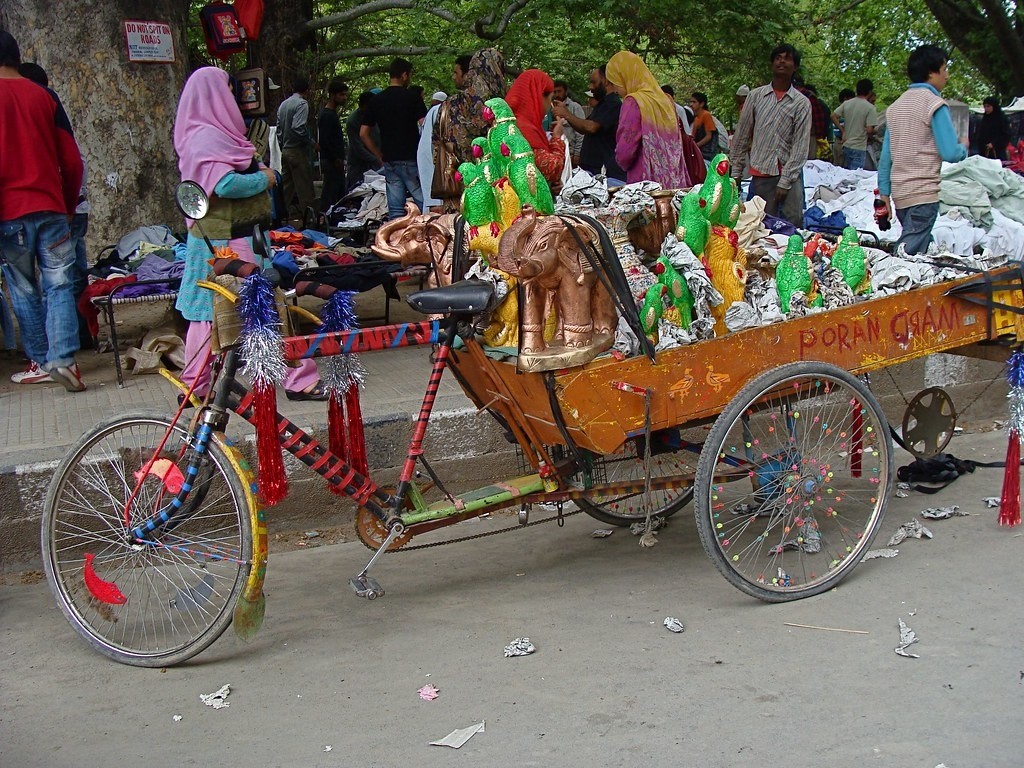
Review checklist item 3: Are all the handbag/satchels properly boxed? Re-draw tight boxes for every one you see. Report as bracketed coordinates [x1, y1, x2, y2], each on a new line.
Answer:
[234, 67, 268, 117]
[677, 116, 707, 184]
[200, 4, 245, 56]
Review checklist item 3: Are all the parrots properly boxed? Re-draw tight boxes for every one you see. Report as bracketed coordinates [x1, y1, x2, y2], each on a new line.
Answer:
[698, 153, 745, 233]
[453, 97, 555, 239]
[776, 235, 813, 310]
[676, 194, 713, 275]
[831, 226, 867, 292]
[639, 283, 669, 338]
[653, 258, 693, 322]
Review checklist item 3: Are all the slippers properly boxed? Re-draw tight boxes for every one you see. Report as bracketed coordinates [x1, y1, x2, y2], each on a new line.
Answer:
[285, 380, 329, 400]
[178, 393, 216, 408]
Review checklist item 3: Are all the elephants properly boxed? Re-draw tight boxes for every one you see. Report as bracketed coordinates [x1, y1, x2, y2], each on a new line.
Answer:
[371, 203, 481, 322]
[496, 205, 618, 351]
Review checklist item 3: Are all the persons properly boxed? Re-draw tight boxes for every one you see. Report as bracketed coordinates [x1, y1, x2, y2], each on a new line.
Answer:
[977, 96, 1014, 166]
[175, 65, 275, 408]
[277, 43, 875, 225]
[0, 31, 91, 393]
[876, 46, 964, 255]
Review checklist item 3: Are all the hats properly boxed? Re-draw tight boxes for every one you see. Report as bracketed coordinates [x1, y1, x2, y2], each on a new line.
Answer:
[432, 91, 448, 102]
[584, 90, 594, 97]
[736, 84, 750, 96]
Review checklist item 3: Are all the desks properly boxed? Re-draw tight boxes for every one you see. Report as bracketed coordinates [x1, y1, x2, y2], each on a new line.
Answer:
[302, 207, 384, 246]
[88, 239, 427, 388]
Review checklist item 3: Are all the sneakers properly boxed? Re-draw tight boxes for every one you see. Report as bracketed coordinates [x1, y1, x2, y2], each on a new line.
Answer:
[11, 359, 55, 383]
[50, 363, 85, 391]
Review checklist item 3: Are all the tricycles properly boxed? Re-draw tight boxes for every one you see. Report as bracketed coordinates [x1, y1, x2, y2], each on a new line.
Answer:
[41, 180, 1024, 667]
